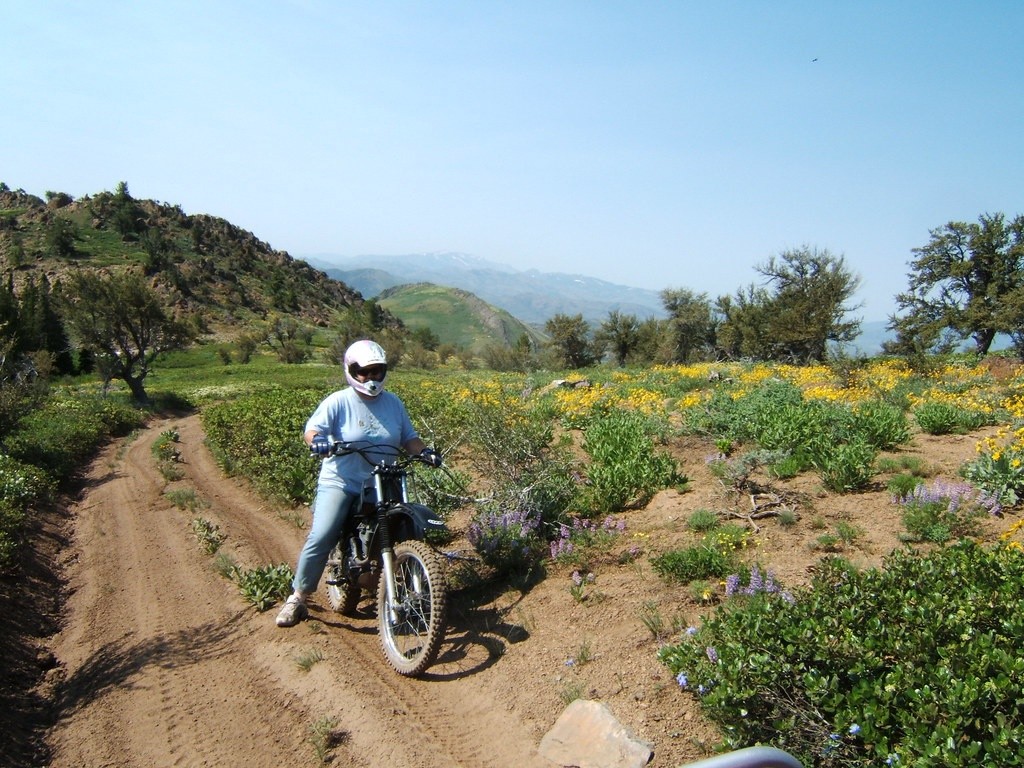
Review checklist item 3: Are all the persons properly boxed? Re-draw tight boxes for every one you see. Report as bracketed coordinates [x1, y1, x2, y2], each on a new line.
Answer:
[275, 339, 442, 629]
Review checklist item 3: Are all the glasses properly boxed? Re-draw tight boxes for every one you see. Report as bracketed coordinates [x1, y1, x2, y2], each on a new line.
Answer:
[353, 367, 382, 375]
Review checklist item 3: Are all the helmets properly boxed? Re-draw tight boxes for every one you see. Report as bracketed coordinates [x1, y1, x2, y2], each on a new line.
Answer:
[342, 340, 389, 397]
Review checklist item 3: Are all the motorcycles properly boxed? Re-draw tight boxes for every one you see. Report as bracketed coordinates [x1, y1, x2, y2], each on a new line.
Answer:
[311, 437, 449, 678]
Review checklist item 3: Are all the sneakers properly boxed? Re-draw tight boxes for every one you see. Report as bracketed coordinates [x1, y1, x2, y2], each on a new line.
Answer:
[276, 596, 309, 626]
